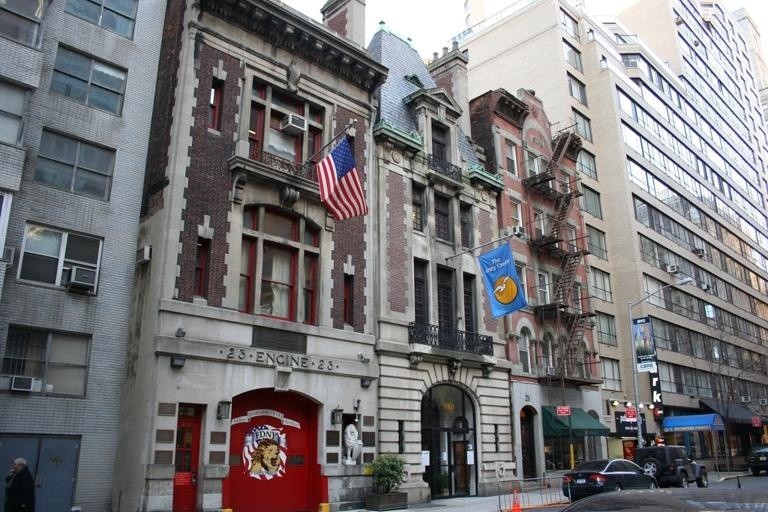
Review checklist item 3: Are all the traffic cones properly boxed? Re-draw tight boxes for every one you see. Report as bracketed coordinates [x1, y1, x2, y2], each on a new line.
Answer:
[511, 487, 522, 511]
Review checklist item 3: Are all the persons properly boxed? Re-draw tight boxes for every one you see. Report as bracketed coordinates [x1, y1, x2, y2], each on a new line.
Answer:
[3, 458, 36, 511]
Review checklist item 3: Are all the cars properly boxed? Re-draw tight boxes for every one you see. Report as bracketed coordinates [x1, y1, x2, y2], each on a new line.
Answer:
[561, 457, 658, 504]
[521, 475, 768, 511]
[747, 447, 767, 475]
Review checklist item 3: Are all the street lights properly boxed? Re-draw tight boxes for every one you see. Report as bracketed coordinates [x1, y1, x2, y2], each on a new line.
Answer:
[626, 276, 693, 449]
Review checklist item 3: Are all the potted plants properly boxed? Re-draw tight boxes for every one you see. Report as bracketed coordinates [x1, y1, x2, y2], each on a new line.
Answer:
[364, 455, 409, 511]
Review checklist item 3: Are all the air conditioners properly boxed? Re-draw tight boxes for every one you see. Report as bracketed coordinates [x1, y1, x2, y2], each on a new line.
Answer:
[69, 266, 97, 290]
[513, 226, 525, 236]
[10, 376, 35, 393]
[281, 113, 307, 136]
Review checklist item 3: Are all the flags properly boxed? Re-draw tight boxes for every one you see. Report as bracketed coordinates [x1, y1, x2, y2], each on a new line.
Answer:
[313, 135, 370, 227]
[478, 241, 527, 321]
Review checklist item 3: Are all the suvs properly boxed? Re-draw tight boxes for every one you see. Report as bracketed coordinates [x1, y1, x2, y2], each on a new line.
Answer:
[633, 444, 709, 488]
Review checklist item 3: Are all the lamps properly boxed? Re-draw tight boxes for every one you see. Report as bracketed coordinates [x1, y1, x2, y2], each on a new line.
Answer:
[332, 404, 344, 425]
[612, 400, 654, 410]
[217, 399, 232, 420]
